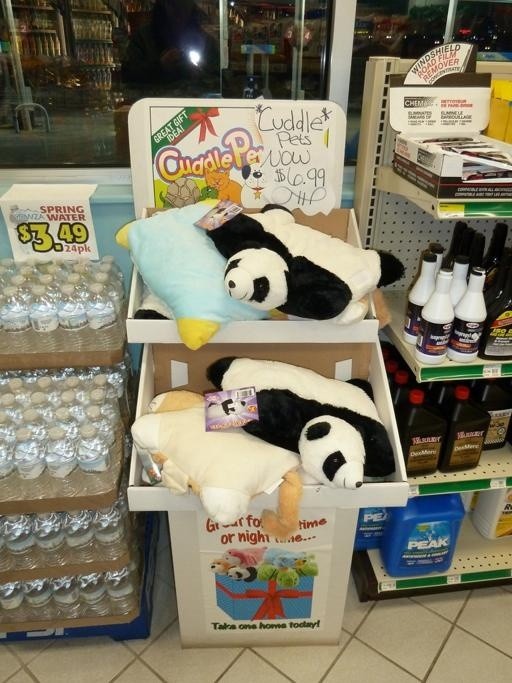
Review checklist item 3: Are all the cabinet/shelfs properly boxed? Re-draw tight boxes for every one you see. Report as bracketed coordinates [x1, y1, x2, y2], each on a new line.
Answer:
[352, 51, 511, 606]
[0, 303, 155, 646]
[117, 95, 416, 652]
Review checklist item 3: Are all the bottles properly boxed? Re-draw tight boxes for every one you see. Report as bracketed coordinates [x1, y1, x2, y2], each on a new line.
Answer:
[402, 219, 510, 364]
[377, 339, 512, 477]
[209, 4, 292, 66]
[1, 350, 140, 621]
[1, 0, 156, 164]
[0, 256, 127, 354]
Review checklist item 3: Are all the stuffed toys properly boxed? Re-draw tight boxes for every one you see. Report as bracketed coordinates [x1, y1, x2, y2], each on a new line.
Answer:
[208, 545, 321, 592]
[206, 204, 405, 319]
[207, 357, 395, 490]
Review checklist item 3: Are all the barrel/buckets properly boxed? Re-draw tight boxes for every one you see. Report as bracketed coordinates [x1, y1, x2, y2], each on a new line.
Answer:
[377, 342, 511, 478]
[353, 493, 464, 578]
[469, 489, 512, 542]
